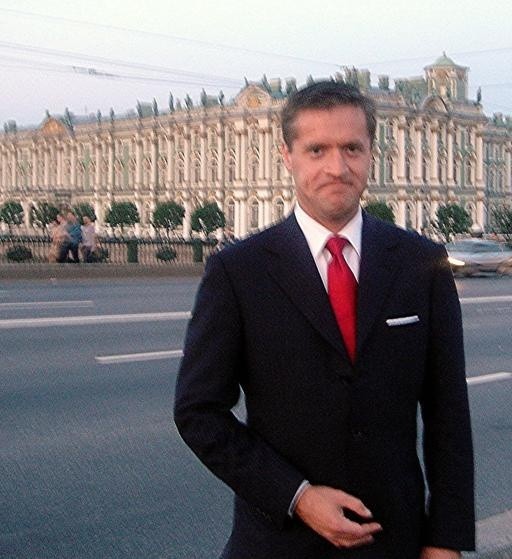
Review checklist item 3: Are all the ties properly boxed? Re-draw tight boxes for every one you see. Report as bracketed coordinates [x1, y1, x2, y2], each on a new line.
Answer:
[324, 237, 358, 366]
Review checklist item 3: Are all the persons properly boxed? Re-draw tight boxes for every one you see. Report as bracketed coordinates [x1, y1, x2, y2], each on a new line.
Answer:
[170, 81, 478, 559]
[48, 213, 66, 262]
[55, 211, 81, 262]
[81, 215, 96, 262]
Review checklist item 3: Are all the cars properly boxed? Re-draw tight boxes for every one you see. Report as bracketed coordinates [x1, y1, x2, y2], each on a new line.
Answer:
[444, 239, 511, 278]
